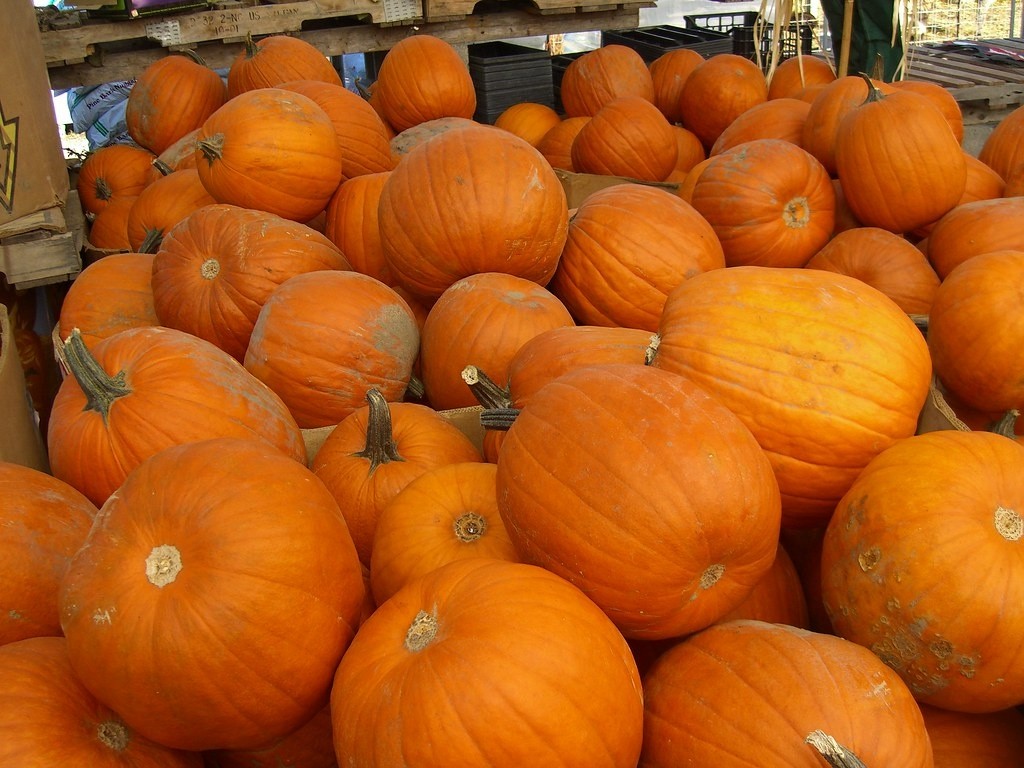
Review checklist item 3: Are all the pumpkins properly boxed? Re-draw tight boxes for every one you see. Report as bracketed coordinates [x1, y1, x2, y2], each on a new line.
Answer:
[0, 28, 1023, 767]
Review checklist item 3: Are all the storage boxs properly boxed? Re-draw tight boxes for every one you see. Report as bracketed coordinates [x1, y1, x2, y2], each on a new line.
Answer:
[687, 10, 815, 77]
[65, 0, 210, 22]
[0, 1, 70, 228]
